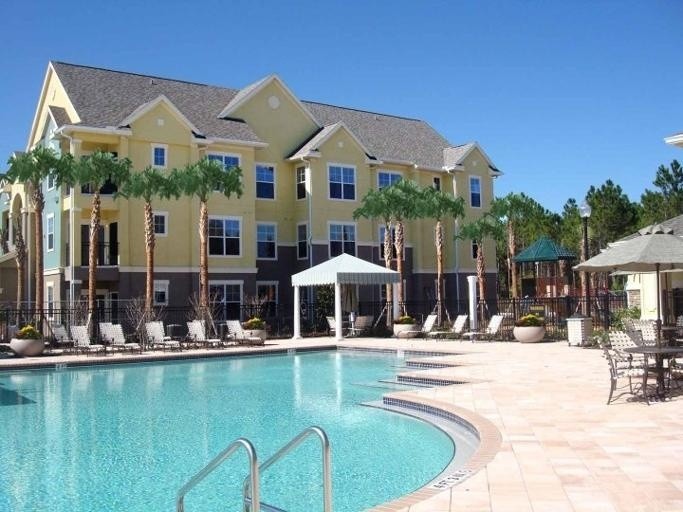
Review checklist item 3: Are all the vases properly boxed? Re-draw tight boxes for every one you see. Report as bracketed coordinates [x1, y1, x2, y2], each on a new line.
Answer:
[8, 337, 44, 357]
[512, 326, 545, 342]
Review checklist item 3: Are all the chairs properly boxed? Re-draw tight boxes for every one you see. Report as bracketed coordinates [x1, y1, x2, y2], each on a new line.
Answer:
[596, 313, 682, 405]
[52, 315, 264, 358]
[321, 308, 507, 347]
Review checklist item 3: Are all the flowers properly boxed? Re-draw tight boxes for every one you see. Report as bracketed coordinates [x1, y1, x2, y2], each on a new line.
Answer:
[513, 313, 544, 326]
[16, 325, 42, 340]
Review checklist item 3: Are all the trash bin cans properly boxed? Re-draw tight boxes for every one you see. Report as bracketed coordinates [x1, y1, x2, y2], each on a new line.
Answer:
[566, 317, 594, 347]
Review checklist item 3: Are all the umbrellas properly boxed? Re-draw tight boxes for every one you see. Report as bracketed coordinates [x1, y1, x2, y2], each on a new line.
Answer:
[608, 268, 683, 327]
[572, 225, 683, 350]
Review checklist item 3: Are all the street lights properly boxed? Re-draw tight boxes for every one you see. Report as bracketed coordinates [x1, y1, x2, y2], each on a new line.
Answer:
[577, 198, 592, 318]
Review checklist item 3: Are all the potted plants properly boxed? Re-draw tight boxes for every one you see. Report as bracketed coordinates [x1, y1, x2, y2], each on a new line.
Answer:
[565, 312, 593, 346]
